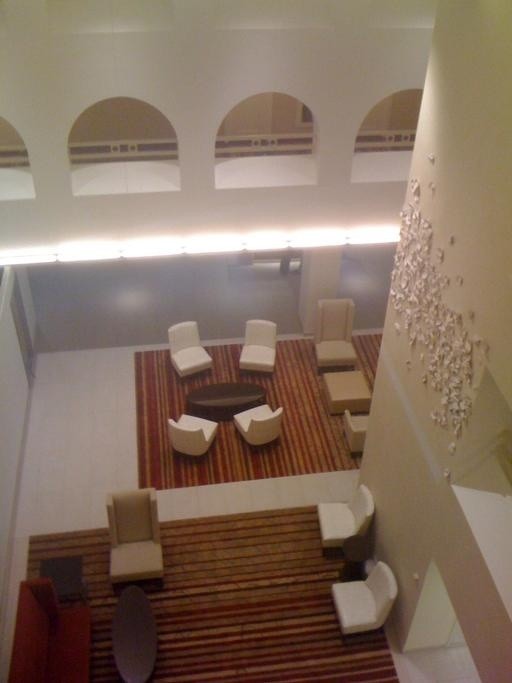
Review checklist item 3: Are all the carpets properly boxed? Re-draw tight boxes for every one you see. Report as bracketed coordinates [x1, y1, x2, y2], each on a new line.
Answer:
[25, 503, 400, 682]
[133, 333, 381, 491]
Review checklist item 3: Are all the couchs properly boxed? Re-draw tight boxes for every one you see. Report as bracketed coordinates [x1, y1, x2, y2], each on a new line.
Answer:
[6, 575, 89, 678]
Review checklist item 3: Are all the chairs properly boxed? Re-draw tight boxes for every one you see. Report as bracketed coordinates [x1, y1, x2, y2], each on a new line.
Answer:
[341, 405, 372, 453]
[101, 485, 171, 583]
[314, 296, 357, 368]
[166, 412, 219, 458]
[165, 320, 213, 378]
[234, 403, 283, 447]
[328, 560, 400, 632]
[315, 483, 375, 546]
[238, 318, 278, 373]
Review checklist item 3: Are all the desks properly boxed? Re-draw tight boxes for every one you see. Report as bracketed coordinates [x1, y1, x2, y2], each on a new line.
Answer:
[185, 381, 267, 421]
[109, 585, 168, 678]
[322, 371, 372, 415]
